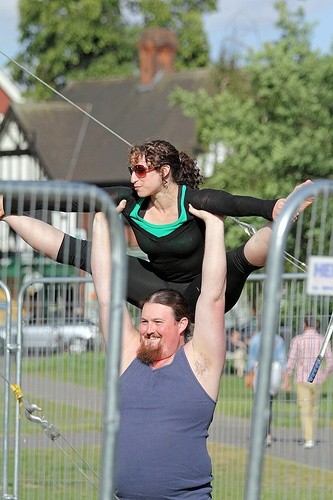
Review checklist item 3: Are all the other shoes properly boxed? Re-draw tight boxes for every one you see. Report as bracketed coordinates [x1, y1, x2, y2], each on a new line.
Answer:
[304, 440, 314, 449]
[265, 438, 272, 447]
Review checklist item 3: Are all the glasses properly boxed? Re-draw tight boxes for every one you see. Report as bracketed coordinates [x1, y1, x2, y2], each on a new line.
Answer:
[128, 165, 161, 178]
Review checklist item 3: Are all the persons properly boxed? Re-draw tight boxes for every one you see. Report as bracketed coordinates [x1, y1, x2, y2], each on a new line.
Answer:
[246, 324, 286, 446]
[91, 199, 228, 500]
[0, 140, 316, 323]
[287, 319, 333, 447]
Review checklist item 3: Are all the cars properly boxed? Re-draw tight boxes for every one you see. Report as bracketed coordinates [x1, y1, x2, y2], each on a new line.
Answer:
[0, 309, 100, 357]
